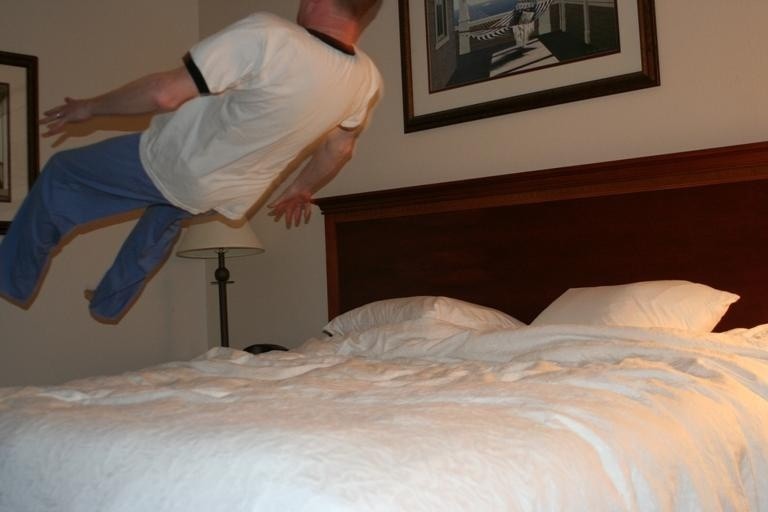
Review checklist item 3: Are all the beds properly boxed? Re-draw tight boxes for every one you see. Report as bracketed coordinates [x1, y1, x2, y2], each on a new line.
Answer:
[0, 140, 767, 511]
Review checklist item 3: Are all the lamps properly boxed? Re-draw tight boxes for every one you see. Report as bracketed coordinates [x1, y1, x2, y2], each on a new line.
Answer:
[175, 210, 266, 348]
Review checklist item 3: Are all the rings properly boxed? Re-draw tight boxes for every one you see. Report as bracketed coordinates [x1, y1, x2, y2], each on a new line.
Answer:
[54, 112, 61, 120]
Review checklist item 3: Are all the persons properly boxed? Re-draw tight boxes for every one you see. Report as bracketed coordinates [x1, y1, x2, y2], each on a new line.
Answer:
[0, 0, 383, 326]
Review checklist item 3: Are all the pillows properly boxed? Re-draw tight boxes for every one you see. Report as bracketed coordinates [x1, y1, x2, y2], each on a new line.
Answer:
[321, 295, 528, 337]
[529, 280, 741, 333]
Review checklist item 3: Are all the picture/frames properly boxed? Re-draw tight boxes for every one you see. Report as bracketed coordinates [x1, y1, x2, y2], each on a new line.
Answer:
[0, 51, 38, 235]
[399, 0, 661, 135]
